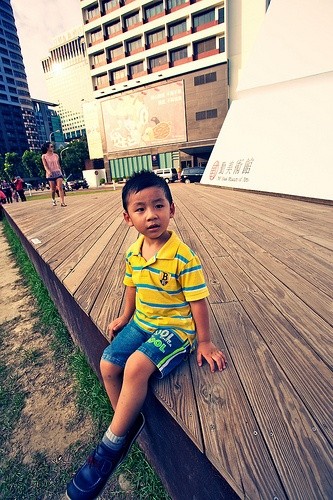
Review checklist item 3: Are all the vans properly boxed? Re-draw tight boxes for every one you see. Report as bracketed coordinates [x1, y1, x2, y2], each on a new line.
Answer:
[181, 166, 206, 184]
[152, 168, 178, 184]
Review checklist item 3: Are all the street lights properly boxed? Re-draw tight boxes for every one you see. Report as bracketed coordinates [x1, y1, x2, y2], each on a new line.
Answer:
[49, 129, 60, 141]
[60, 146, 72, 178]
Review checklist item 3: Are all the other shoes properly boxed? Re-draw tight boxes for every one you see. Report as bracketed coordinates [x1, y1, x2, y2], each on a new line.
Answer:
[52, 200, 57, 206]
[65, 411, 145, 500]
[61, 203, 67, 207]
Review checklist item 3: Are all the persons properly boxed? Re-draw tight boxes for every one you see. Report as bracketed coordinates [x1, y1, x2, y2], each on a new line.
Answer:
[0, 176, 27, 204]
[64, 169, 229, 500]
[40, 141, 67, 207]
